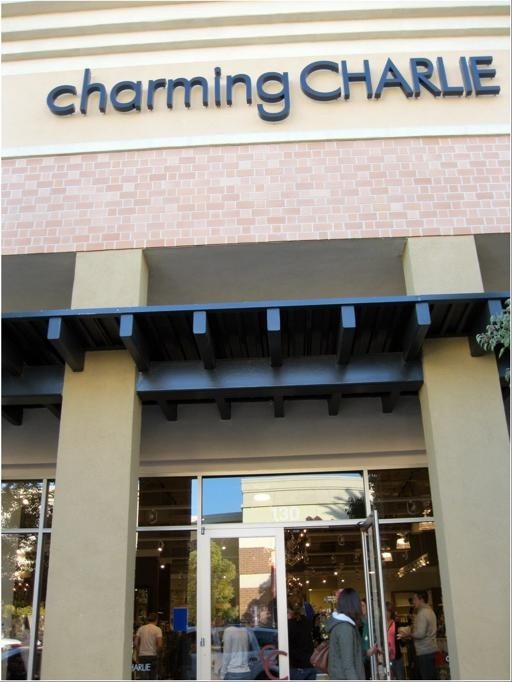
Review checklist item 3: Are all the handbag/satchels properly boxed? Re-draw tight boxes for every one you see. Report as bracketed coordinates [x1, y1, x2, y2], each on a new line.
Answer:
[310, 640, 329, 670]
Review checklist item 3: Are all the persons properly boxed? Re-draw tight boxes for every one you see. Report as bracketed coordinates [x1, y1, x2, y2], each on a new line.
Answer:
[396, 590, 441, 679]
[219, 604, 253, 680]
[359, 598, 371, 680]
[132, 611, 214, 680]
[324, 586, 379, 680]
[269, 596, 317, 679]
[384, 600, 396, 679]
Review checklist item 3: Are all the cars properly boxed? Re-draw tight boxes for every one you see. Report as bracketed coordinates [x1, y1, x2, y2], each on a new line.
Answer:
[188, 627, 279, 680]
